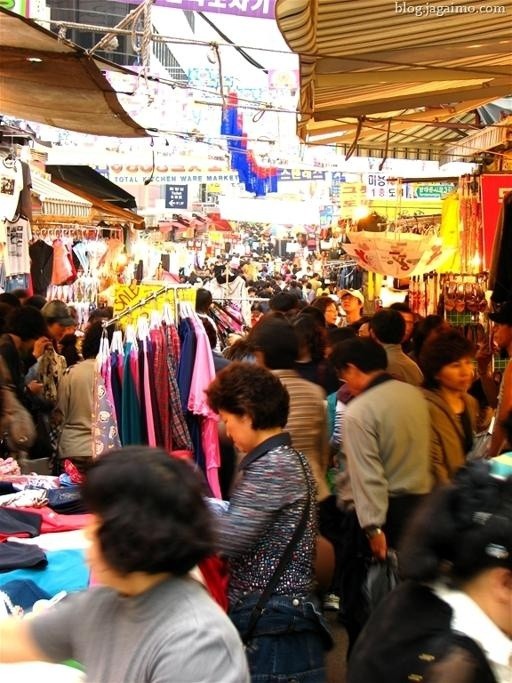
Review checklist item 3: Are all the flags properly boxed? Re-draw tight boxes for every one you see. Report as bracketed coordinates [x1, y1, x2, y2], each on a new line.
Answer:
[219, 93, 281, 198]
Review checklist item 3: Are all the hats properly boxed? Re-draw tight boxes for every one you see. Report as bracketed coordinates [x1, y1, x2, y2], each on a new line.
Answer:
[336, 288, 364, 307]
[41, 299, 75, 326]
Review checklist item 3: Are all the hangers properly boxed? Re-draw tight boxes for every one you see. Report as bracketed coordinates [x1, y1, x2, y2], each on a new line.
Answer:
[35, 226, 121, 243]
[97, 282, 199, 372]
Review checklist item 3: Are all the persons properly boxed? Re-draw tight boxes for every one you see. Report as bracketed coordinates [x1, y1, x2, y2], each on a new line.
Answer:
[0, 225, 512, 682]
